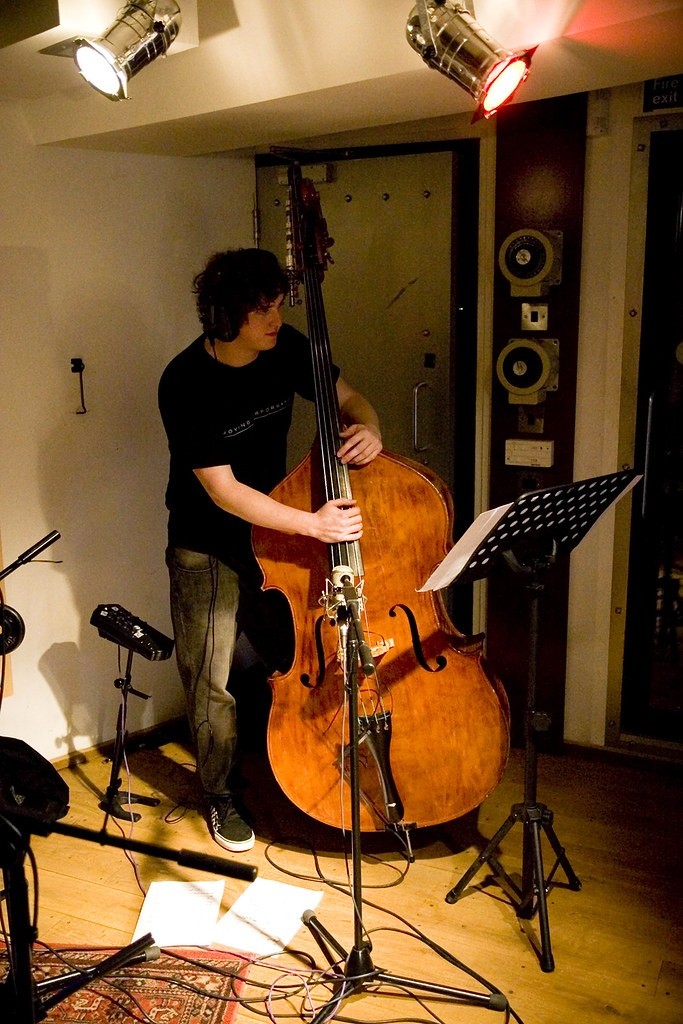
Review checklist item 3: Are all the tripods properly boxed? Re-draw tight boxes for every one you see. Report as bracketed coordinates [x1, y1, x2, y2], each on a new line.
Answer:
[445, 468, 639, 972]
[305, 592, 507, 1024]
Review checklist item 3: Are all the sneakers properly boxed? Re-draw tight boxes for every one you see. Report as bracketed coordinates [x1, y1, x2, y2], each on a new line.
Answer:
[208, 794, 255, 852]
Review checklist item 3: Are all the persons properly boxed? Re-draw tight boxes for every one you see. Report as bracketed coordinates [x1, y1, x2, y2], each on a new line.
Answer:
[159, 249, 384, 851]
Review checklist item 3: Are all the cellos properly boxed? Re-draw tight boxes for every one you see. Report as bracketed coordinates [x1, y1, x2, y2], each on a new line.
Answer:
[262, 173, 516, 842]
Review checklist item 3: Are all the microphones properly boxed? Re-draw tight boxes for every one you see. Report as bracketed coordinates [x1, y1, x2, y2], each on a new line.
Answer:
[332, 565, 354, 648]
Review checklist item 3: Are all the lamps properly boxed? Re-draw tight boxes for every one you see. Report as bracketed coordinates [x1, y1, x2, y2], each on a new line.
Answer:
[406, 0, 539, 126]
[73, 0, 182, 102]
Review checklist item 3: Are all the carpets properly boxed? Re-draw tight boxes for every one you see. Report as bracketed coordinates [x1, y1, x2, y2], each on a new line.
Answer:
[0, 938, 254, 1024]
